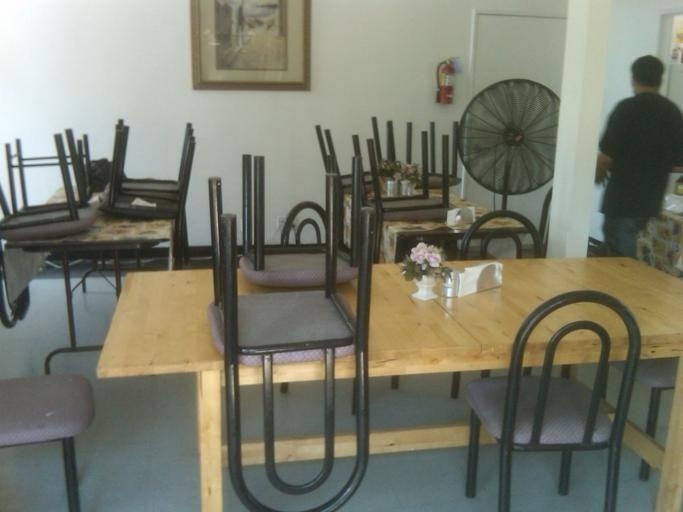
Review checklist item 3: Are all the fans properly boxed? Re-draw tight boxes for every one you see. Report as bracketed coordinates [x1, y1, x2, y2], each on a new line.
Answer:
[455, 78, 560, 211]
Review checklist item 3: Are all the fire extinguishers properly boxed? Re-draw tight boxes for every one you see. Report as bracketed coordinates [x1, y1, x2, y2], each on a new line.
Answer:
[436, 57, 459, 104]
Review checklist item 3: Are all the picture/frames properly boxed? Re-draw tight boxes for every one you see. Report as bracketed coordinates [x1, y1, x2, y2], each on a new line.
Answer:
[190, 1, 310, 90]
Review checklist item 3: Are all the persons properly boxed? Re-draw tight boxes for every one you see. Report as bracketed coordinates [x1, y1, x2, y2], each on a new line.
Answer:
[594, 54, 682, 260]
